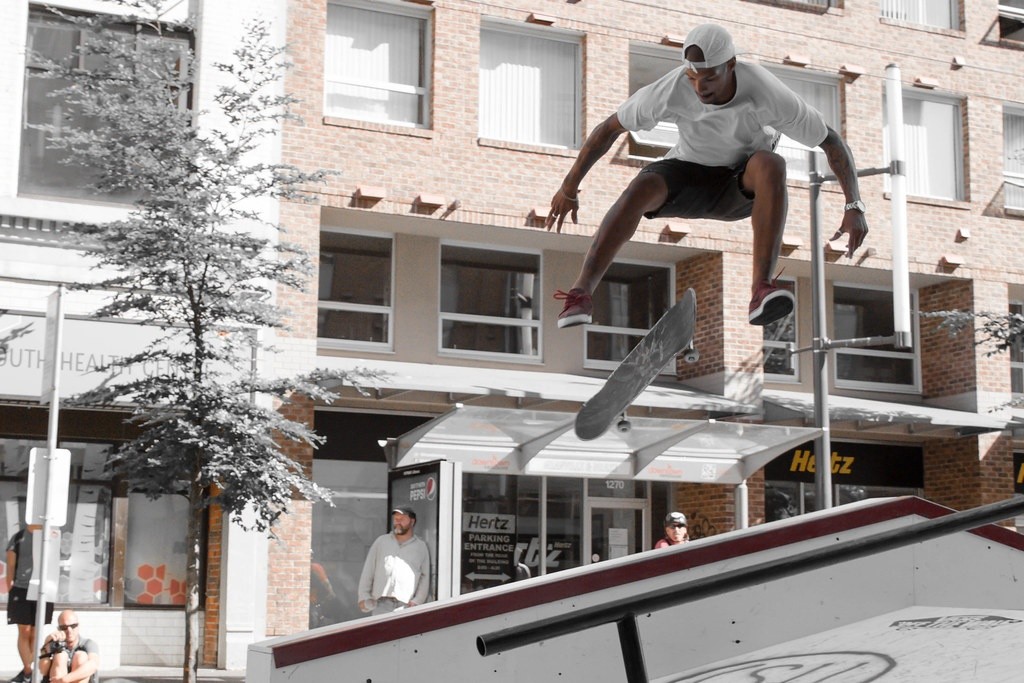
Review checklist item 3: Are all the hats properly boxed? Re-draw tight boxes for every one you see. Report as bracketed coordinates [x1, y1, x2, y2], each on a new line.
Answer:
[681, 23, 746, 70]
[392, 505, 416, 515]
[664, 511, 688, 527]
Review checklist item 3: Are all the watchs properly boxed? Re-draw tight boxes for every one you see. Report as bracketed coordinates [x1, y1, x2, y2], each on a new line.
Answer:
[843, 200, 866, 213]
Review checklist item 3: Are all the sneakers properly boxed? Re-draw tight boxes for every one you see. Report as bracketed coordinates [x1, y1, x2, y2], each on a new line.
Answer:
[553, 288, 593, 329]
[748, 284, 796, 326]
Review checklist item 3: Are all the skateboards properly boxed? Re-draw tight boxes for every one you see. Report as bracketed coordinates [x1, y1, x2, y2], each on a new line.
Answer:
[574, 288, 700, 441]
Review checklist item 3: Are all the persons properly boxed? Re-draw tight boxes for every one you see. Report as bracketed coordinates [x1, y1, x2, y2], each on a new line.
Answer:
[40, 609, 101, 683]
[356, 505, 430, 619]
[654, 511, 690, 550]
[545, 22, 869, 329]
[6, 524, 44, 683]
[309, 547, 333, 631]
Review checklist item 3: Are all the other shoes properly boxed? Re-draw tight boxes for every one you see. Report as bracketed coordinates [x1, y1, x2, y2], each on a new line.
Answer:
[9, 669, 32, 683]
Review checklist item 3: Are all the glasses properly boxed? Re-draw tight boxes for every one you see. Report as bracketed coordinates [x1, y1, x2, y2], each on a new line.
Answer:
[666, 524, 685, 529]
[58, 624, 79, 630]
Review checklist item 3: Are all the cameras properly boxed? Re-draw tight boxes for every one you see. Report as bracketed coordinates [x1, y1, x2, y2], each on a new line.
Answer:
[50, 641, 67, 653]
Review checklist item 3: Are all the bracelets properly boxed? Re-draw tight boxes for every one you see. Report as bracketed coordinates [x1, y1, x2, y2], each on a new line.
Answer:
[560, 186, 578, 202]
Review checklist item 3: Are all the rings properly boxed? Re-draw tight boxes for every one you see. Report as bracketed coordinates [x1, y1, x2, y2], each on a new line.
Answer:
[836, 229, 843, 236]
[552, 213, 558, 218]
